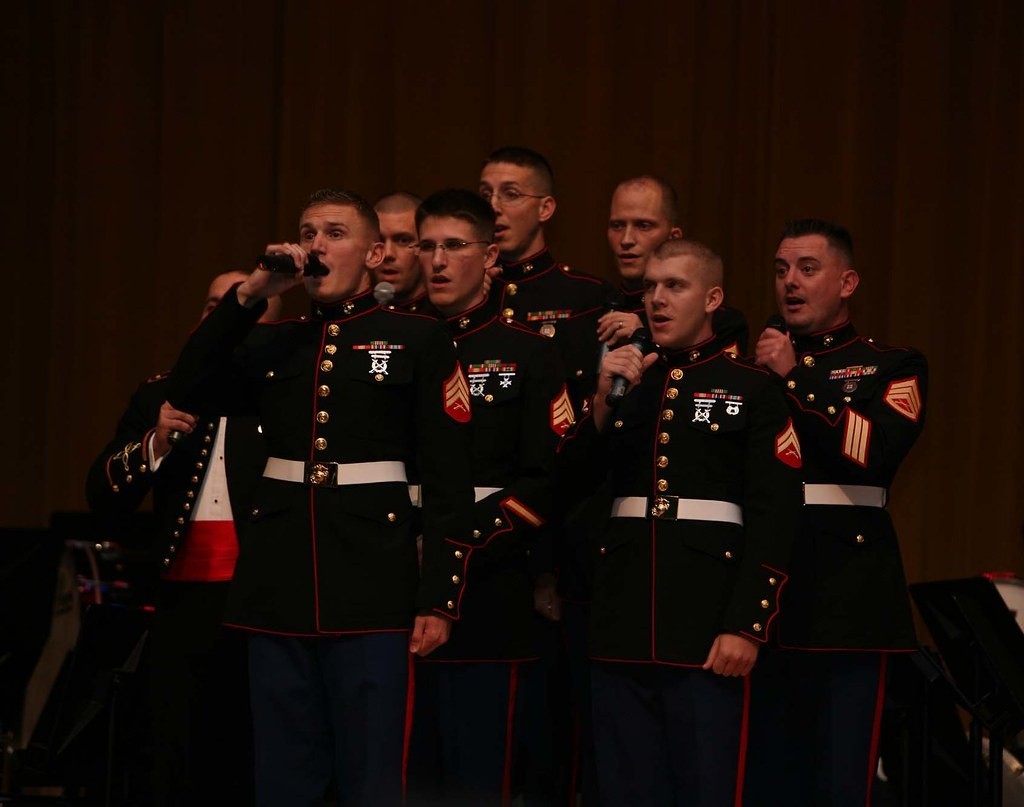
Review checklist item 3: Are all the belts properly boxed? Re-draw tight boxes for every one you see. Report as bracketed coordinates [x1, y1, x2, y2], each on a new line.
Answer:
[802, 482, 886, 507]
[610, 496, 745, 527]
[262, 457, 408, 486]
[408, 485, 504, 508]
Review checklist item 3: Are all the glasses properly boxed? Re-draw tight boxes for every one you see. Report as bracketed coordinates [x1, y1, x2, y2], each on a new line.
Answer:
[408, 240, 490, 255]
[482, 191, 546, 202]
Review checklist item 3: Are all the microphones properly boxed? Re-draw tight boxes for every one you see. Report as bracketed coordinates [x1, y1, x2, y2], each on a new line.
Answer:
[374, 281, 396, 307]
[257, 253, 320, 277]
[167, 430, 185, 446]
[765, 315, 788, 371]
[605, 327, 652, 408]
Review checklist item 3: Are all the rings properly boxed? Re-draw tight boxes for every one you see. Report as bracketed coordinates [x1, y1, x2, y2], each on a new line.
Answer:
[619, 321, 624, 329]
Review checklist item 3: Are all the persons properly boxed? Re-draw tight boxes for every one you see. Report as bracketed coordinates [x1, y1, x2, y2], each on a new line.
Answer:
[382, 189, 580, 805]
[363, 146, 749, 807]
[563, 234, 806, 807]
[733, 219, 928, 807]
[162, 189, 473, 806]
[84, 268, 269, 807]
